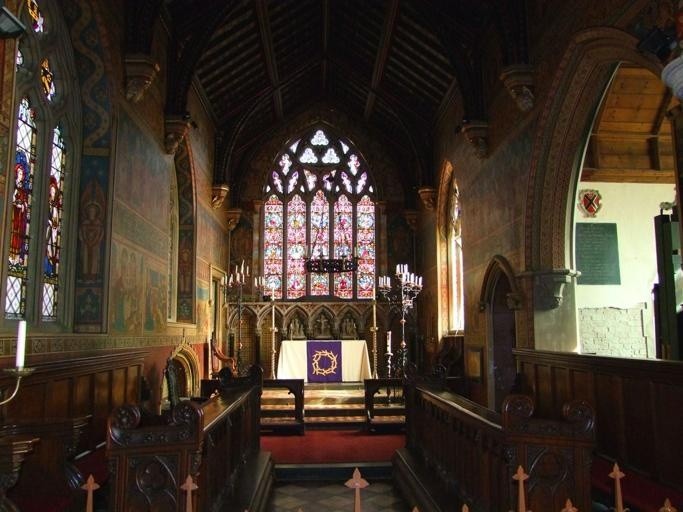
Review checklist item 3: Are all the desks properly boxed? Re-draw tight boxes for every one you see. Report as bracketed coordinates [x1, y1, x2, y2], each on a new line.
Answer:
[274, 338, 373, 385]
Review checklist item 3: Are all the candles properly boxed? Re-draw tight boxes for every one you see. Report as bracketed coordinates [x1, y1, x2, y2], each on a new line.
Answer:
[372, 303, 377, 329]
[219, 259, 275, 301]
[385, 330, 392, 354]
[12, 319, 26, 369]
[371, 263, 423, 301]
[271, 304, 276, 328]
